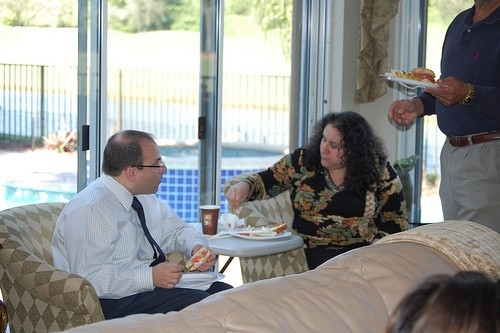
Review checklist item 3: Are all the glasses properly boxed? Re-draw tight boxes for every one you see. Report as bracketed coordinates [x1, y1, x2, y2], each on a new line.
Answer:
[131, 160, 163, 171]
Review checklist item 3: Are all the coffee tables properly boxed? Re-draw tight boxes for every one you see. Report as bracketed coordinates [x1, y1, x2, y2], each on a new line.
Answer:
[184, 220, 304, 274]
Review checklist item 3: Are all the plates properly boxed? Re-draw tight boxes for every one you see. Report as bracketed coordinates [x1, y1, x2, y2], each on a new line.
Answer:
[175, 272, 225, 287]
[386, 77, 440, 88]
[229, 230, 292, 240]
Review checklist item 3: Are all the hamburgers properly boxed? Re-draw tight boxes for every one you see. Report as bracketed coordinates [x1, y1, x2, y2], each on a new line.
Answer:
[185, 247, 210, 271]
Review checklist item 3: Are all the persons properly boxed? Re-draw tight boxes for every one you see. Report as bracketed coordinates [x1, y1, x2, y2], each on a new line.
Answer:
[384, 271, 500, 333]
[51, 130, 233, 320]
[388, 0, 500, 234]
[224, 111, 413, 271]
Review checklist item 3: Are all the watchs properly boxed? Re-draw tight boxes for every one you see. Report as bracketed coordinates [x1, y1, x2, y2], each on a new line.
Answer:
[460, 83, 474, 105]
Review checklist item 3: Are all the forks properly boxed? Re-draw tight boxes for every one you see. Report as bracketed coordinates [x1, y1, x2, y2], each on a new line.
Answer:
[398, 82, 425, 89]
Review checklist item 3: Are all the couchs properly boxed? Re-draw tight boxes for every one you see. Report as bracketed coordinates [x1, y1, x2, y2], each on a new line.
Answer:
[0, 200, 200, 333]
[61, 220, 500, 332]
[223, 173, 309, 286]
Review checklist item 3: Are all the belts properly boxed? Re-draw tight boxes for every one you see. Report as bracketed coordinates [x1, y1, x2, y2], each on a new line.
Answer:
[449, 131, 500, 147]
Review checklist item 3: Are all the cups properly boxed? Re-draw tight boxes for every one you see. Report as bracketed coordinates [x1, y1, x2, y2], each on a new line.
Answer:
[199, 204, 220, 236]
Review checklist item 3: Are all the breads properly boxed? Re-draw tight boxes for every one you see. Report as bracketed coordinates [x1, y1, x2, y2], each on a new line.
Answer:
[410, 67, 437, 78]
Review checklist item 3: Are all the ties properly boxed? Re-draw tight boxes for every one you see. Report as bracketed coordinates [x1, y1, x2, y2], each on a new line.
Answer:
[131, 196, 166, 266]
